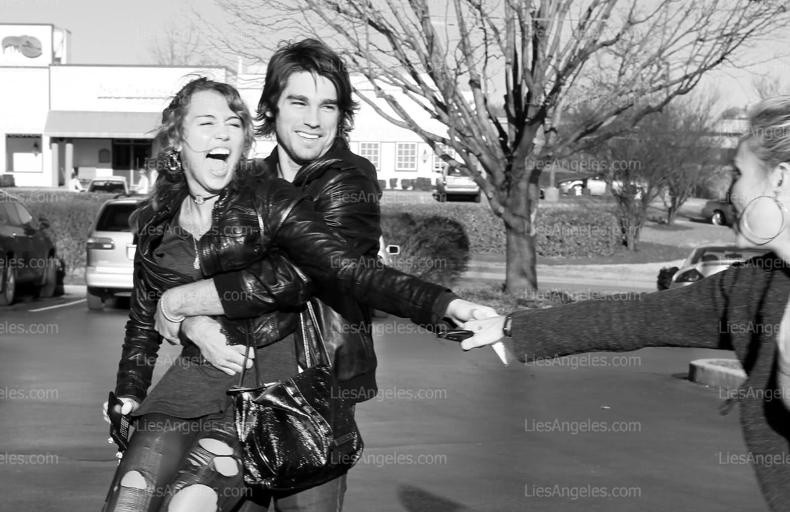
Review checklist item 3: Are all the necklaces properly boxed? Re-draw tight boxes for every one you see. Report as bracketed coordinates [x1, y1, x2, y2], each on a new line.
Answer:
[189, 208, 209, 269]
[185, 191, 220, 205]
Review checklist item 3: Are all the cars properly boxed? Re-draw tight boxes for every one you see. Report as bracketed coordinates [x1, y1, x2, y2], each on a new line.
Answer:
[85, 198, 137, 310]
[560, 167, 652, 200]
[671, 238, 770, 293]
[80, 173, 135, 199]
[702, 196, 734, 227]
[0, 191, 66, 305]
[432, 159, 481, 201]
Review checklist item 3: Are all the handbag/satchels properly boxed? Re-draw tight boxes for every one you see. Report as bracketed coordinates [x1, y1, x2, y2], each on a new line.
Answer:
[226, 363, 364, 496]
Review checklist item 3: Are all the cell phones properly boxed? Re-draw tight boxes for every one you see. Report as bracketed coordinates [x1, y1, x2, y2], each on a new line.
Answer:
[444, 330, 485, 349]
[106, 391, 131, 450]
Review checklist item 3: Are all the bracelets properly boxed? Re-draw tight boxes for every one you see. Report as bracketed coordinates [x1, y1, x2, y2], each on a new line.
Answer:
[503, 309, 512, 338]
[157, 291, 185, 325]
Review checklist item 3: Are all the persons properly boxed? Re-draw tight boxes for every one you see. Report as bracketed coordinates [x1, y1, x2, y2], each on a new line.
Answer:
[134, 168, 150, 194]
[103, 68, 502, 512]
[143, 158, 160, 191]
[154, 37, 383, 512]
[459, 94, 790, 512]
